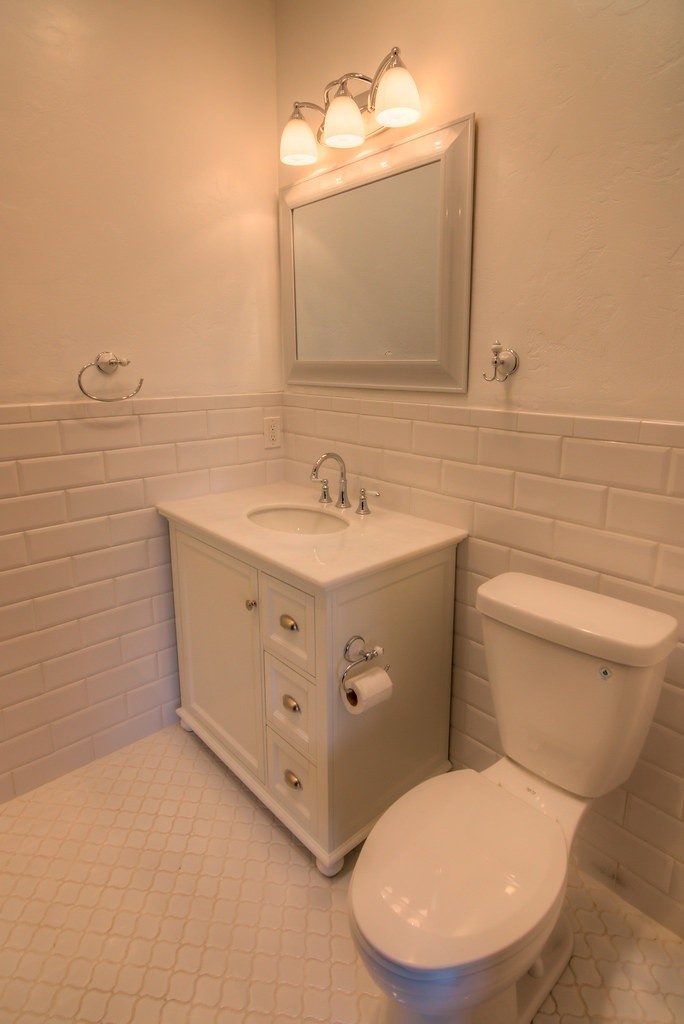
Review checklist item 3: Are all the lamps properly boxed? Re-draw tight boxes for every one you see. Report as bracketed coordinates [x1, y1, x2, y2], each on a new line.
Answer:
[280, 47, 420, 166]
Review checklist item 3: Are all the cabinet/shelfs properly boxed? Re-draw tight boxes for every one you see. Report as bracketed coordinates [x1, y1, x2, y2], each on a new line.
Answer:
[167, 519, 316, 837]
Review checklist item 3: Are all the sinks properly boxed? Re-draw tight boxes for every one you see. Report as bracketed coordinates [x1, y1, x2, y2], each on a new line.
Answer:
[246, 503, 351, 536]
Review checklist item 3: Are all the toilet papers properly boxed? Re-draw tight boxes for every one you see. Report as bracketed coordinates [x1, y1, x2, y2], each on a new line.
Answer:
[339, 665, 394, 716]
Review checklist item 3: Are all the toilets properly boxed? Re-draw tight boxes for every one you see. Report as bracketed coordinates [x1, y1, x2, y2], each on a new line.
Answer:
[344, 571, 681, 1017]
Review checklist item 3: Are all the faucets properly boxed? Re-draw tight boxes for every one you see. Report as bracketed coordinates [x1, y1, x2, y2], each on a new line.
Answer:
[310, 452, 352, 509]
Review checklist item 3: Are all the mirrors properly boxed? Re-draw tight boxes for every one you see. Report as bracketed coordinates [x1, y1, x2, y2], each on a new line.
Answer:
[278, 113, 475, 394]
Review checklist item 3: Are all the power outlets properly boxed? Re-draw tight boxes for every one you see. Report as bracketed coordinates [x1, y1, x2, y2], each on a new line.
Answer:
[261, 414, 282, 449]
